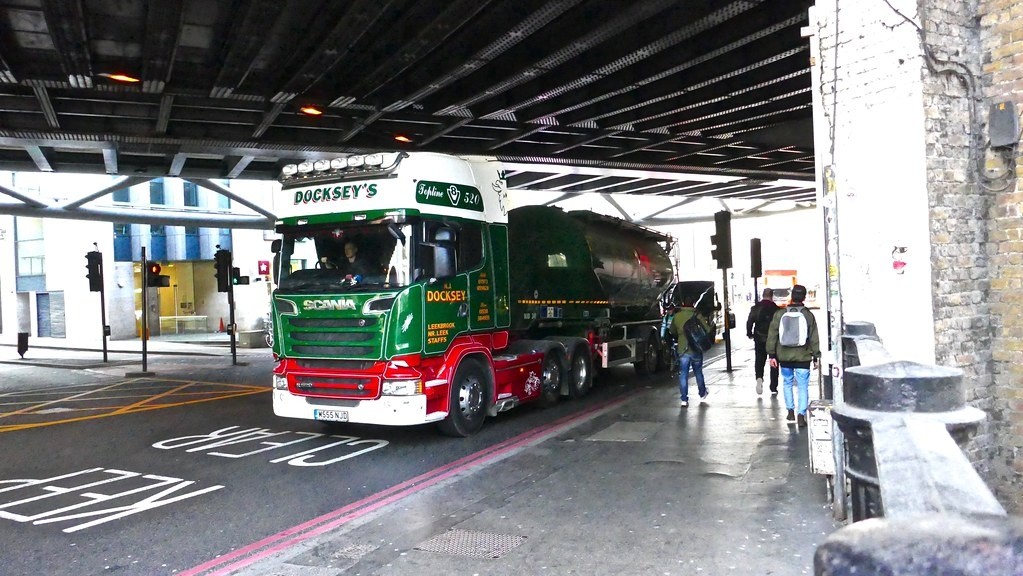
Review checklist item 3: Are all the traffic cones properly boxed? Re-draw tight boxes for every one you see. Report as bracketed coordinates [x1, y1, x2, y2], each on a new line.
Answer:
[219, 317, 225, 332]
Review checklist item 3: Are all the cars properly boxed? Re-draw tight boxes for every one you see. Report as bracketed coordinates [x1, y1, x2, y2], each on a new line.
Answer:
[661, 279, 726, 344]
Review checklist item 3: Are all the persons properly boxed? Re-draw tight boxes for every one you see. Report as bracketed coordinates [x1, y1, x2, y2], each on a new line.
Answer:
[766, 284, 821, 427]
[747, 288, 780, 396]
[321, 240, 371, 285]
[669, 296, 710, 408]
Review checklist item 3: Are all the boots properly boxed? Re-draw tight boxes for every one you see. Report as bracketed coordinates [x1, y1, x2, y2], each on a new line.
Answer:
[798, 413, 807, 426]
[786, 410, 795, 421]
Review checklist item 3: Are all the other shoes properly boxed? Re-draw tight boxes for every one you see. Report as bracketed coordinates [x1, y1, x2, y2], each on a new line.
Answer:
[700, 387, 709, 399]
[756, 378, 763, 395]
[681, 401, 690, 406]
[772, 390, 778, 394]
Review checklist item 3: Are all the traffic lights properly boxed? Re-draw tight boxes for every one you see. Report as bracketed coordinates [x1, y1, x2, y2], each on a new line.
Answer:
[709, 211, 733, 270]
[85, 251, 101, 292]
[231, 267, 240, 285]
[146, 261, 170, 288]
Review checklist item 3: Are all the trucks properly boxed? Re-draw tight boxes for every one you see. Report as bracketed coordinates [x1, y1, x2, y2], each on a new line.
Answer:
[766, 275, 794, 307]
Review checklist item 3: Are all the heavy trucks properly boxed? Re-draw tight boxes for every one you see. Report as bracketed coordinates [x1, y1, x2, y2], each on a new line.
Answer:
[266, 146, 662, 436]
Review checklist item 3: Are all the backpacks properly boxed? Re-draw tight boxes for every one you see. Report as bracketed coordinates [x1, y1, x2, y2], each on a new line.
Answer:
[779, 306, 808, 347]
[682, 311, 712, 353]
